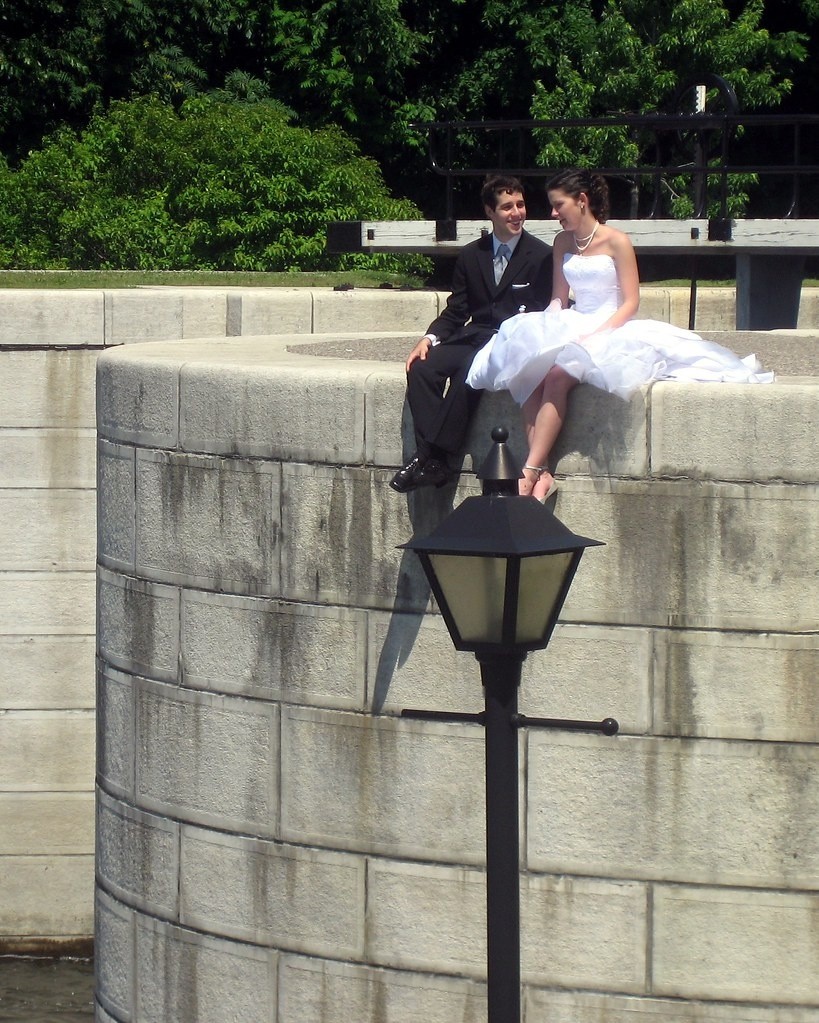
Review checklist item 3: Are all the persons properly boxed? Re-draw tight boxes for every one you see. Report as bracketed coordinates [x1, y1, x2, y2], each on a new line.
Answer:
[464, 164, 776, 505]
[389, 173, 554, 493]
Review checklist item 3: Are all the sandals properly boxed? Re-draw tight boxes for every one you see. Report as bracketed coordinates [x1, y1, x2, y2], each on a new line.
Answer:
[531, 465, 558, 504]
[518, 466, 542, 495]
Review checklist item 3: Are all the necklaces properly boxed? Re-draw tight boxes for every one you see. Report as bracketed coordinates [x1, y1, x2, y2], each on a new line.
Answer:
[572, 221, 599, 252]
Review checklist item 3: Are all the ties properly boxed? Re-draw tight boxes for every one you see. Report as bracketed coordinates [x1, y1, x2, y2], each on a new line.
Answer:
[493, 244, 512, 286]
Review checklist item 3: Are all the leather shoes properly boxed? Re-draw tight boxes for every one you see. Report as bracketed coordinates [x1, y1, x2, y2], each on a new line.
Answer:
[412, 464, 448, 489]
[389, 452, 424, 493]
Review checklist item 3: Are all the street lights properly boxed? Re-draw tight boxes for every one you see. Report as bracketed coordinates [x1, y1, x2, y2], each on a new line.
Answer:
[397, 424, 610, 1023]
